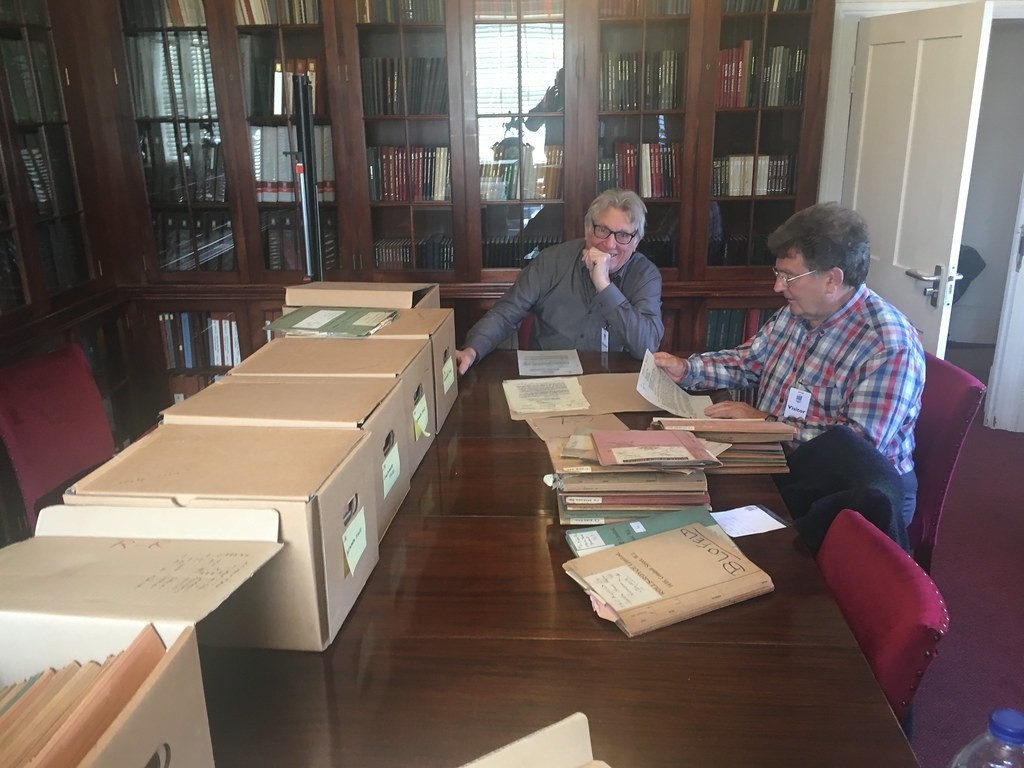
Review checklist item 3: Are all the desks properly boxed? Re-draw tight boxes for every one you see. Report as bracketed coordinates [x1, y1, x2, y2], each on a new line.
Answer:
[205, 349, 922, 768]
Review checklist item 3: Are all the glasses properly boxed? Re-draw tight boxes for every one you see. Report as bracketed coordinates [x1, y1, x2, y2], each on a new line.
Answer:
[591, 215, 639, 244]
[772, 265, 831, 288]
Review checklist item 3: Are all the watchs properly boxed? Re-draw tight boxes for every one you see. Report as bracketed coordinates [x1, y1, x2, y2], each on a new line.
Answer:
[765, 414, 778, 422]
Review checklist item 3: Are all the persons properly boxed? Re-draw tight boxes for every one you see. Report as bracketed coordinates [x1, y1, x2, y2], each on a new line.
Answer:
[652, 201, 926, 527]
[455, 188, 665, 375]
[525, 68, 564, 145]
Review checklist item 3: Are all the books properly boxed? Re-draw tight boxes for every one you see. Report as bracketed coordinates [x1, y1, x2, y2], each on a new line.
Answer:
[479, 145, 563, 200]
[156, 312, 241, 369]
[709, 233, 768, 266]
[144, 142, 236, 271]
[362, 57, 449, 115]
[636, 233, 672, 268]
[240, 34, 326, 117]
[355, 0, 445, 23]
[374, 234, 454, 269]
[367, 144, 452, 201]
[713, 152, 798, 196]
[125, 0, 206, 27]
[599, 0, 690, 18]
[600, 49, 688, 111]
[234, 0, 319, 26]
[482, 236, 562, 268]
[249, 123, 337, 270]
[598, 141, 681, 198]
[722, 0, 812, 12]
[716, 39, 806, 106]
[556, 418, 797, 638]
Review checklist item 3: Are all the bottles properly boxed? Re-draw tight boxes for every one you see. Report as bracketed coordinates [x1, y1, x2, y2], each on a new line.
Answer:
[944, 706, 1024, 768]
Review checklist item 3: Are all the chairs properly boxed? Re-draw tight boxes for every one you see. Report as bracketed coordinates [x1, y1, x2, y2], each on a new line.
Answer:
[0, 343, 118, 547]
[905, 351, 987, 577]
[814, 509, 950, 739]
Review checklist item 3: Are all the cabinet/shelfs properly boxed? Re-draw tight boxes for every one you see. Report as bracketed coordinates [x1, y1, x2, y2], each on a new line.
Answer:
[0, 0, 836, 546]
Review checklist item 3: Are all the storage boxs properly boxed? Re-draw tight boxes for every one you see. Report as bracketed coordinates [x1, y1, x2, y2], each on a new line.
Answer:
[273, 305, 458, 435]
[0, 504, 285, 768]
[281, 280, 439, 317]
[214, 337, 436, 479]
[155, 374, 411, 545]
[61, 424, 380, 653]
